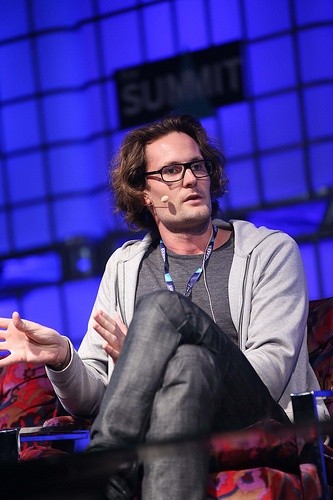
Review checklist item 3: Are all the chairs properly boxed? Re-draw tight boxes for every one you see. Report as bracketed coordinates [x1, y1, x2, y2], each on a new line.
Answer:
[96, 296, 333, 500]
[0, 339, 88, 497]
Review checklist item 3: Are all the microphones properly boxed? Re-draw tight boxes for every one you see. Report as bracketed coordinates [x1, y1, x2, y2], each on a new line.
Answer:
[143, 195, 170, 213]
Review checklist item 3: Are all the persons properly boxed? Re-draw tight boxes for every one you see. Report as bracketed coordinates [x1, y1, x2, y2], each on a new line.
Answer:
[1, 114, 331, 499]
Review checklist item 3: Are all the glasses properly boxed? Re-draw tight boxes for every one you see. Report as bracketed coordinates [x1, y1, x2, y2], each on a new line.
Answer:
[138, 159, 213, 183]
